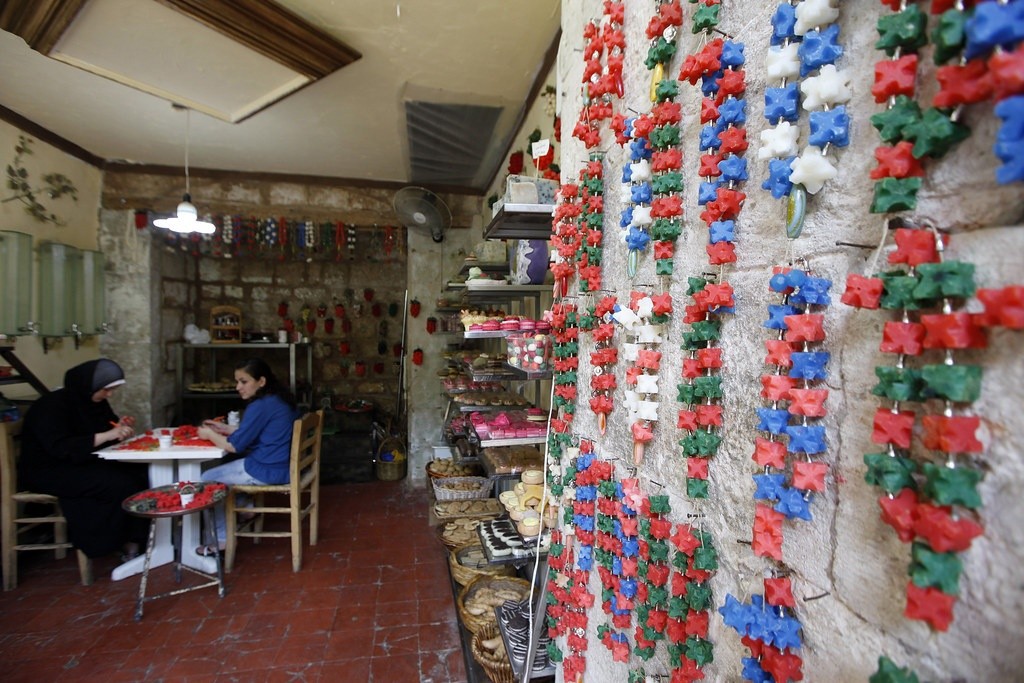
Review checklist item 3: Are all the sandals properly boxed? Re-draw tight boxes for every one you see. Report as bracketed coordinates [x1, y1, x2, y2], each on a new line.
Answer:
[195, 544, 227, 558]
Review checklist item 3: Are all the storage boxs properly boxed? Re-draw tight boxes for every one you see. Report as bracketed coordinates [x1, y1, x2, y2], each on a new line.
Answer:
[320, 407, 381, 486]
[429, 441, 452, 459]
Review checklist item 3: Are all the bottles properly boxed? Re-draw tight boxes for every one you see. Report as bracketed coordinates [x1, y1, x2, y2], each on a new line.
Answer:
[217, 317, 238, 338]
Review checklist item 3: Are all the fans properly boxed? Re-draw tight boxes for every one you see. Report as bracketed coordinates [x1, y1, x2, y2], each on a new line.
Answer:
[393, 186, 452, 243]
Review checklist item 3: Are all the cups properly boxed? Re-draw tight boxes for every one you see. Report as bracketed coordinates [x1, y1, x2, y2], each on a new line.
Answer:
[278, 330, 287, 343]
[180, 493, 194, 508]
[228, 412, 239, 425]
[159, 435, 172, 451]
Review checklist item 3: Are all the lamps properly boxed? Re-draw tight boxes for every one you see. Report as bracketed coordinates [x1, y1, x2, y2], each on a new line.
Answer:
[154, 103, 216, 234]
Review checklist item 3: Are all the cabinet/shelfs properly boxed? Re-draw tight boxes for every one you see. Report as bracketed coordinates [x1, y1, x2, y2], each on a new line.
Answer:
[426, 203, 559, 683]
[177, 342, 313, 410]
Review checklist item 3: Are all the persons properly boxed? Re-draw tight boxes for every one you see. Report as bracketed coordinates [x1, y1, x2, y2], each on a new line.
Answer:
[196, 356, 302, 556]
[18, 358, 151, 562]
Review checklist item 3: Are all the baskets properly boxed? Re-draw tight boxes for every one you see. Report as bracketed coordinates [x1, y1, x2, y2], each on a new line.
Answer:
[425, 460, 535, 682]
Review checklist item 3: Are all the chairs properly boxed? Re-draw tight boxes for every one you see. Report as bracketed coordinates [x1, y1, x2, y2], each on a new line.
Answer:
[0, 420, 92, 591]
[225, 410, 323, 574]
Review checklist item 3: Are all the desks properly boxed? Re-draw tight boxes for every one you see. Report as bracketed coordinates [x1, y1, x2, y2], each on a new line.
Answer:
[122, 482, 229, 622]
[91, 428, 229, 581]
